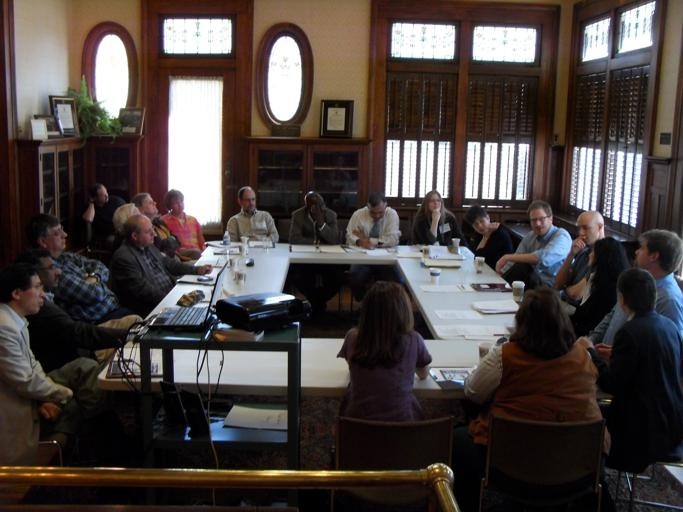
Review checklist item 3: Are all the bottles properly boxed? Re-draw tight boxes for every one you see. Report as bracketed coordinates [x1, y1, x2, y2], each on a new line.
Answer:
[222, 230, 231, 245]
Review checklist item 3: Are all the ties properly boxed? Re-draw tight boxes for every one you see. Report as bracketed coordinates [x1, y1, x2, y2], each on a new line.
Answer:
[370, 218, 380, 239]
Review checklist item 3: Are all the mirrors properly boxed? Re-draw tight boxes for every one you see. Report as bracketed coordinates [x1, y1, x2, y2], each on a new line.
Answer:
[255, 21, 314, 128]
[81, 20, 139, 124]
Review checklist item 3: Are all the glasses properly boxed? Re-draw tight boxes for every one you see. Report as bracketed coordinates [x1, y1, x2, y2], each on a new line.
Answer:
[530, 215, 549, 222]
[40, 263, 57, 271]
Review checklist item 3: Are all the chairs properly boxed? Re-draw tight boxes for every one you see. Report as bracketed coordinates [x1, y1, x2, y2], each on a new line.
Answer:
[277, 218, 343, 314]
[327, 413, 455, 512]
[479, 411, 606, 512]
[336, 219, 366, 314]
[398, 218, 412, 245]
[81, 216, 115, 266]
[614, 457, 683, 511]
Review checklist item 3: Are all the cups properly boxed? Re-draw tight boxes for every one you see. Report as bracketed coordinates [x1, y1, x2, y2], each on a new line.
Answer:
[474, 256, 486, 274]
[511, 280, 525, 302]
[452, 237, 460, 247]
[228, 236, 249, 285]
[476, 342, 493, 359]
[428, 267, 440, 285]
[422, 245, 432, 258]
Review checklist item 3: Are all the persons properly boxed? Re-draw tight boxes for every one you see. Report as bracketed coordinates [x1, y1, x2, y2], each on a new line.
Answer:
[338, 281, 431, 460]
[463, 288, 611, 476]
[464, 205, 513, 270]
[226, 186, 279, 243]
[288, 190, 343, 314]
[496, 200, 573, 293]
[556, 211, 683, 473]
[346, 190, 401, 301]
[81, 184, 213, 314]
[2, 214, 150, 468]
[410, 190, 465, 246]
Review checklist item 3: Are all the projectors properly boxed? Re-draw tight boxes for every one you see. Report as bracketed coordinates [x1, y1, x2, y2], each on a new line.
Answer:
[216, 292, 313, 335]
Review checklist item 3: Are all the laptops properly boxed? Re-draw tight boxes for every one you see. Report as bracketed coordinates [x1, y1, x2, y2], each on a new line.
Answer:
[147, 260, 229, 332]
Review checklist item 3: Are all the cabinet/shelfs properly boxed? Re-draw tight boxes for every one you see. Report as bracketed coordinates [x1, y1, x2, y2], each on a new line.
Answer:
[17, 138, 83, 255]
[137, 317, 304, 507]
[83, 132, 144, 214]
[503, 227, 521, 251]
[244, 136, 375, 244]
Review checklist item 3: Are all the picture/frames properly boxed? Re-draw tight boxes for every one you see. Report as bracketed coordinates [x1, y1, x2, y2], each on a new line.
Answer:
[319, 99, 354, 139]
[48, 94, 81, 138]
[118, 106, 146, 136]
[33, 113, 62, 139]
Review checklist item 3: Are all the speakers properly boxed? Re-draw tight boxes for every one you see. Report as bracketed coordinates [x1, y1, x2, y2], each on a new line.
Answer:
[160, 381, 187, 435]
[179, 389, 209, 435]
